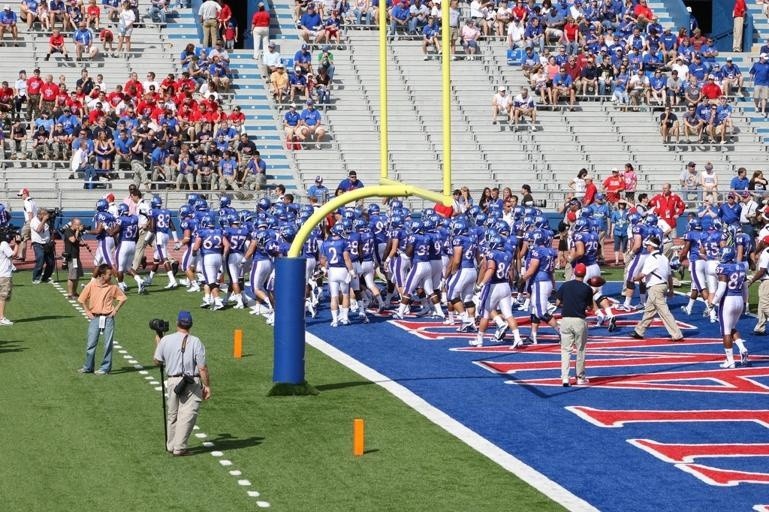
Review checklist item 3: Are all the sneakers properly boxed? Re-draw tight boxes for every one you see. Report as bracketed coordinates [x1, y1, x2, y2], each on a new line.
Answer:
[167, 447, 187, 456]
[719, 360, 736, 369]
[94, 369, 108, 376]
[577, 378, 590, 384]
[740, 349, 749, 366]
[561, 378, 570, 387]
[78, 368, 88, 373]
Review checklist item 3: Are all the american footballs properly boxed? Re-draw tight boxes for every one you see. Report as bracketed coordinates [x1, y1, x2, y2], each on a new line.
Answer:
[586, 276, 606, 286]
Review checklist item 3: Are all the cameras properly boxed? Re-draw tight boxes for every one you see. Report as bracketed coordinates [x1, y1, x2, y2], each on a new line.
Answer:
[79, 225, 91, 234]
[62, 253, 70, 270]
[149, 319, 169, 332]
[174, 373, 195, 396]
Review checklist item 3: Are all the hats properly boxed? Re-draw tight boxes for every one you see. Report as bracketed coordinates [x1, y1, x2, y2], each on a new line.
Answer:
[257, 2, 328, 109]
[742, 191, 749, 198]
[434, 0, 732, 92]
[4, 5, 11, 10]
[317, 176, 323, 183]
[17, 189, 29, 196]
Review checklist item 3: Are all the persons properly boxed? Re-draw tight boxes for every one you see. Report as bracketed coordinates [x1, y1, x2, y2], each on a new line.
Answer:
[555, 264, 594, 388]
[152, 309, 210, 458]
[1, 1, 769, 375]
